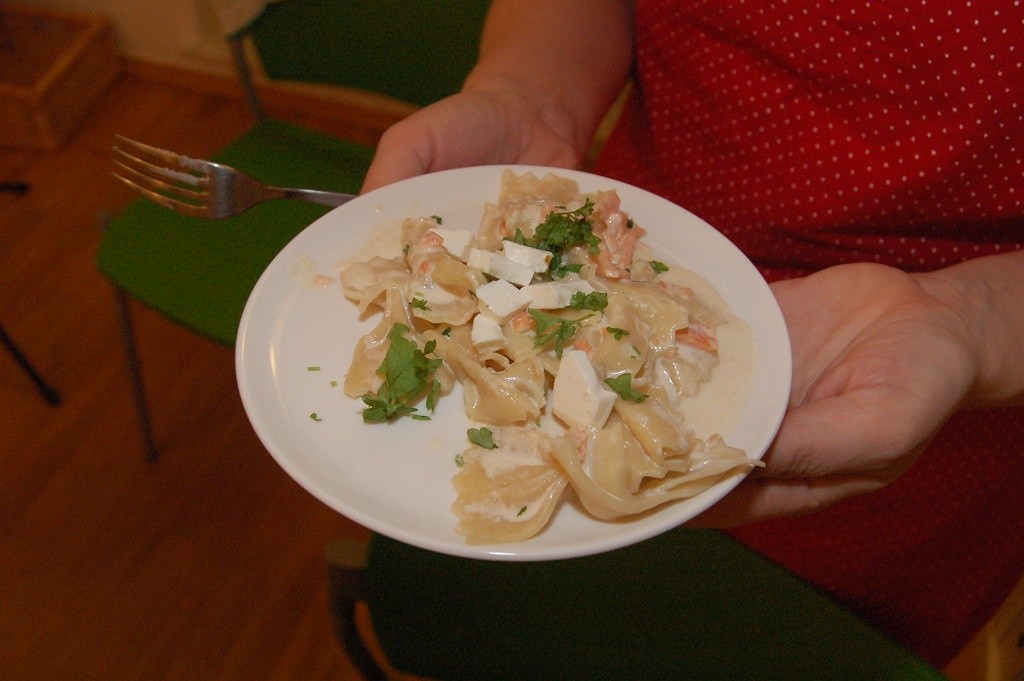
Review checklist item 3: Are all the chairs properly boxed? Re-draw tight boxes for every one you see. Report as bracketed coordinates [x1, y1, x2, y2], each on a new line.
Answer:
[97, 0, 494, 459]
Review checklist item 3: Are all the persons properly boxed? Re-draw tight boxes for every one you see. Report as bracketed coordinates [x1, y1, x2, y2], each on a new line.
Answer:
[358, 0, 1024, 673]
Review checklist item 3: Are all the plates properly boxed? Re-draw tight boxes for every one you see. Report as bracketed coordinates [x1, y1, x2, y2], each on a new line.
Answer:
[235, 164, 794, 563]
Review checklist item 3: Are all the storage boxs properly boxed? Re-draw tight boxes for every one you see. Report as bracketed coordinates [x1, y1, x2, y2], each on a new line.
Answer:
[0, 4, 124, 153]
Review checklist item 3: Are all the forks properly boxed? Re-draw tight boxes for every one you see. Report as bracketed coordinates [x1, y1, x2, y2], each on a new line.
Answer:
[109, 134, 358, 218]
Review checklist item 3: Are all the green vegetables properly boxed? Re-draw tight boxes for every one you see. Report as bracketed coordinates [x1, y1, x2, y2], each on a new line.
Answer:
[307, 197, 670, 469]
